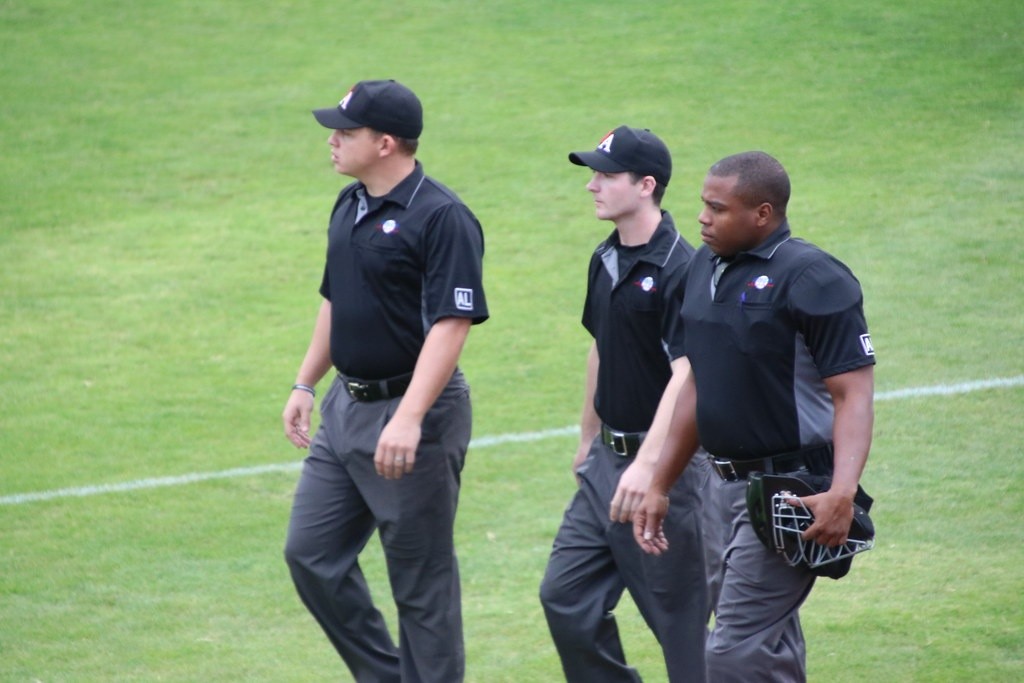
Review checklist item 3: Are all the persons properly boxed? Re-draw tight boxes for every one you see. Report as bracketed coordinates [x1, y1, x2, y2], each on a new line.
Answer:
[540, 126, 707, 683]
[632, 152, 876, 682]
[282, 80, 490, 683]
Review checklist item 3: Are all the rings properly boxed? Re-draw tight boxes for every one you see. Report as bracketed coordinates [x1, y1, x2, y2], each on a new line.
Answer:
[395, 457, 403, 461]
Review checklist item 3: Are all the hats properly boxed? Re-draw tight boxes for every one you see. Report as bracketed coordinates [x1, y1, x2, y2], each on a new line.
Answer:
[568, 124, 672, 186]
[312, 78, 423, 140]
[747, 478, 824, 555]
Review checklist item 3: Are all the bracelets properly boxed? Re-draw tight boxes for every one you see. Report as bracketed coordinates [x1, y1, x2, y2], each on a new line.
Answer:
[293, 384, 315, 397]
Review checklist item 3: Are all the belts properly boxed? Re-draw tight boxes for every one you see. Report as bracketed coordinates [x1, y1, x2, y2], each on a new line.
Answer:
[603, 424, 641, 459]
[708, 444, 834, 484]
[337, 371, 411, 404]
